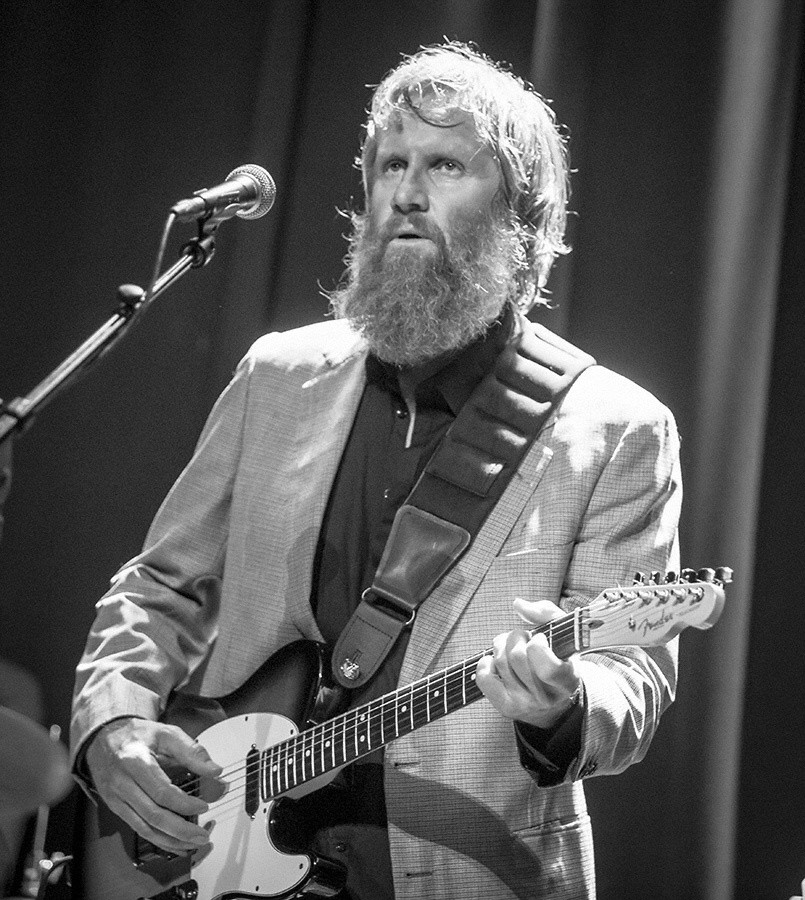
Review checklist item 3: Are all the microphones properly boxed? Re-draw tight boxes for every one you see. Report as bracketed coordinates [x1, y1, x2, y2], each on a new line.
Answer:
[169, 162, 277, 225]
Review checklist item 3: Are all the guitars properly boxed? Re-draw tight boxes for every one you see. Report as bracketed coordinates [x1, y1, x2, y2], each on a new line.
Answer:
[67, 565, 736, 900]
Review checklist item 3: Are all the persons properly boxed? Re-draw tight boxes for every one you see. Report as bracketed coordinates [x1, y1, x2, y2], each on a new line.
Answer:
[69, 42, 682, 900]
